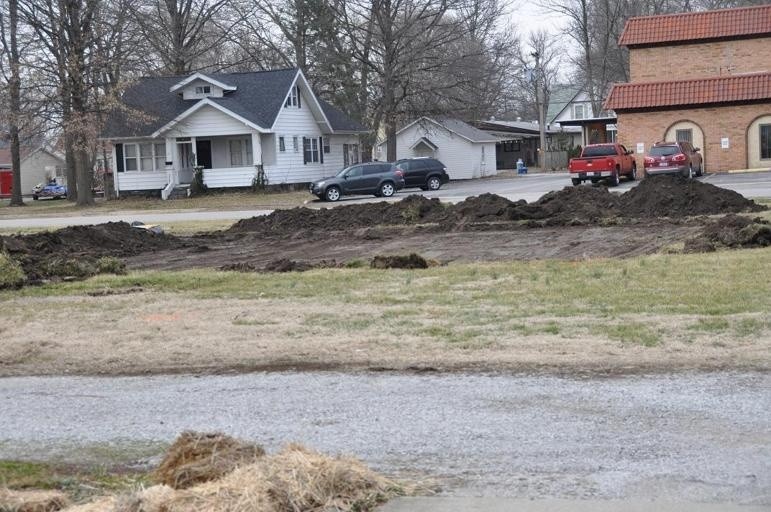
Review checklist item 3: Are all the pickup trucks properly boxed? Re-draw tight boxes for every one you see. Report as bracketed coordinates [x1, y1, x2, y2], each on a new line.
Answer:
[569, 141, 636, 186]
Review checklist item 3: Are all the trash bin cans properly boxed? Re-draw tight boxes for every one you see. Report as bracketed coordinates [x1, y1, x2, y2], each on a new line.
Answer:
[516, 162, 524, 174]
[103, 173, 113, 201]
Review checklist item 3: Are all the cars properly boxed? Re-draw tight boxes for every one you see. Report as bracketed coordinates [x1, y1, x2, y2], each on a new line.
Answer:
[30, 182, 66, 201]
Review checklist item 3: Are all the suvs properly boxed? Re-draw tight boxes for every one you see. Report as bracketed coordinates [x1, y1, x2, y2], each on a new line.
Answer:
[310, 160, 404, 201]
[393, 156, 449, 190]
[643, 141, 703, 178]
